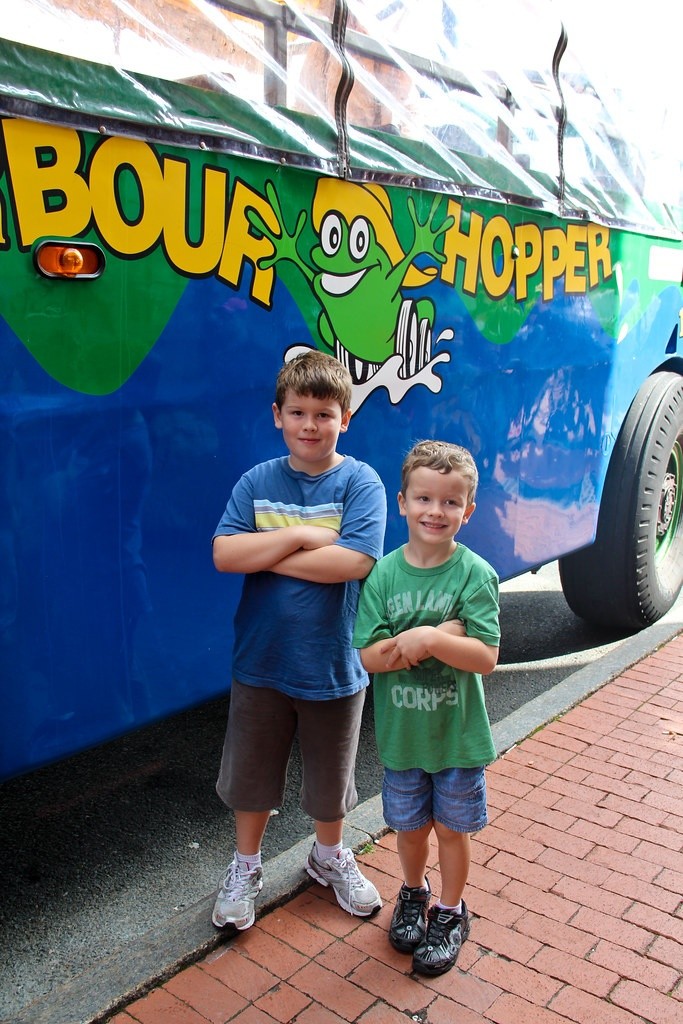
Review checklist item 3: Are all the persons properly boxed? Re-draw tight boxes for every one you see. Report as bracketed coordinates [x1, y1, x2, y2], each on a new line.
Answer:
[197, 350, 388, 936]
[351, 439, 505, 975]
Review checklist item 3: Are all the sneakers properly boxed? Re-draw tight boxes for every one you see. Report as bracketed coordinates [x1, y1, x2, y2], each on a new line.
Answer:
[388, 874, 471, 976]
[304, 839, 382, 917]
[210, 855, 265, 932]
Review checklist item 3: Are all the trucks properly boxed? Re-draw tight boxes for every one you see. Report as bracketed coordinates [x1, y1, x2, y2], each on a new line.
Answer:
[0, 0, 683, 786]
